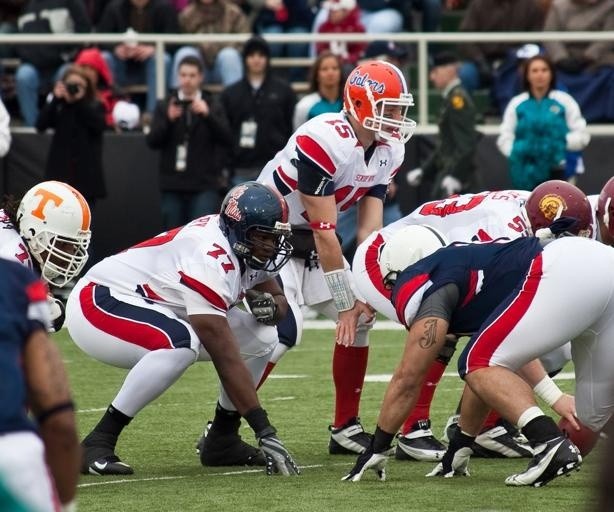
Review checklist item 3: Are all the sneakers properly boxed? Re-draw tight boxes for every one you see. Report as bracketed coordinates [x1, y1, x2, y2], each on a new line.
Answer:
[394, 420, 449, 463]
[505, 435, 584, 489]
[199, 431, 267, 468]
[470, 425, 536, 460]
[80, 445, 134, 477]
[443, 415, 528, 443]
[328, 418, 397, 456]
[194, 421, 213, 450]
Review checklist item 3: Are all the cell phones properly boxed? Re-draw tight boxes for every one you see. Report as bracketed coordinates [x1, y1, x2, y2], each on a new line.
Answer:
[176, 100, 192, 111]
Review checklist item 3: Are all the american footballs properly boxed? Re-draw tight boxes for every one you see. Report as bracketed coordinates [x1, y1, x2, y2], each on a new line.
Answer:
[558, 413, 600, 458]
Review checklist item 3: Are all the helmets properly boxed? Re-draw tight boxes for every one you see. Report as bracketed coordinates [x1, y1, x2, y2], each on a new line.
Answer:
[342, 60, 417, 146]
[596, 177, 614, 237]
[378, 224, 445, 292]
[217, 179, 295, 275]
[15, 180, 92, 288]
[526, 178, 593, 237]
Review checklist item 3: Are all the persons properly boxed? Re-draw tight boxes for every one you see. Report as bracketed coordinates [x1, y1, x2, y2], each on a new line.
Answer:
[341, 225, 614, 488]
[317, 0, 366, 81]
[434, 0, 470, 59]
[74, 46, 132, 130]
[357, 41, 408, 69]
[387, 135, 439, 219]
[195, 59, 417, 454]
[218, 35, 299, 193]
[95, 0, 173, 129]
[169, 0, 246, 92]
[252, 0, 311, 82]
[352, 180, 595, 464]
[32, 66, 109, 209]
[16, 1, 91, 128]
[146, 55, 232, 232]
[1, 180, 93, 335]
[444, 174, 614, 458]
[496, 55, 591, 191]
[406, 52, 483, 204]
[292, 52, 348, 134]
[1, 257, 82, 512]
[544, 0, 614, 126]
[65, 181, 300, 477]
[456, 0, 548, 115]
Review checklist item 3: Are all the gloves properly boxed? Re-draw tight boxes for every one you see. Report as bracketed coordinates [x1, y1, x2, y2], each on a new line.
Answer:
[341, 428, 396, 483]
[425, 431, 476, 479]
[247, 426, 302, 478]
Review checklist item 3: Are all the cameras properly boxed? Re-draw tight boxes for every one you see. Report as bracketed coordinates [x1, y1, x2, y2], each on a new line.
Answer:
[66, 84, 79, 95]
